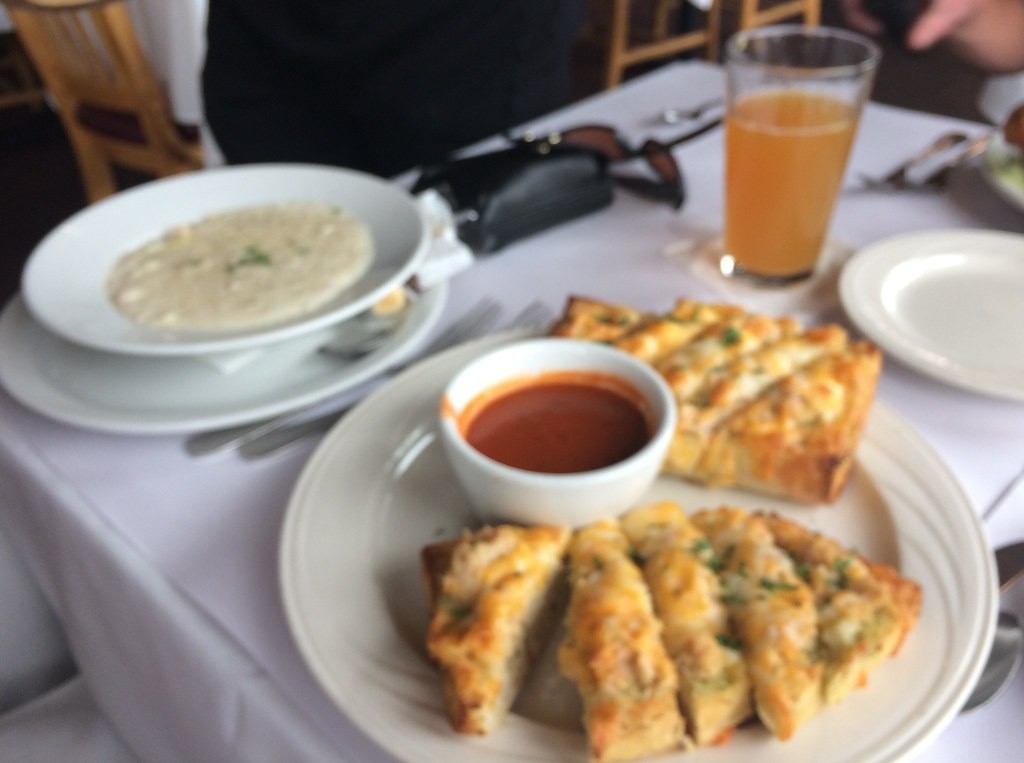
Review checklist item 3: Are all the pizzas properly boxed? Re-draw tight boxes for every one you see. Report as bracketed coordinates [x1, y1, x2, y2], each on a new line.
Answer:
[427, 512, 922, 759]
[549, 295, 879, 502]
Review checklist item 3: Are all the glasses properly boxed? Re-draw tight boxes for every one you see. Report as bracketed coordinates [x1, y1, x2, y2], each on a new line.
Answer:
[411, 126, 685, 210]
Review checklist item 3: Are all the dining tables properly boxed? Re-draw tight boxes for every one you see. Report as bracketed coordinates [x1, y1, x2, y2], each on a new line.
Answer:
[0, 60, 1024, 763]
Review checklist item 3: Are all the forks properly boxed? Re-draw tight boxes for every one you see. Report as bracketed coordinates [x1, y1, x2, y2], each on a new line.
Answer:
[237, 298, 561, 461]
[890, 139, 991, 190]
[857, 133, 966, 189]
[181, 299, 501, 456]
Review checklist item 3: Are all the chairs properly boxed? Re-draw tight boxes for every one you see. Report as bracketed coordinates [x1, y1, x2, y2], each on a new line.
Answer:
[0, 0, 204, 205]
[580, 0, 823, 91]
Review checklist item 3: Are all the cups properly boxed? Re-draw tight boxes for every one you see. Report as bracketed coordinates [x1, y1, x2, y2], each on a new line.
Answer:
[722, 25, 879, 289]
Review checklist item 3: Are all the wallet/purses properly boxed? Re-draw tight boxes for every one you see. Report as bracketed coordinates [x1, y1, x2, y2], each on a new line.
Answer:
[445, 141, 613, 259]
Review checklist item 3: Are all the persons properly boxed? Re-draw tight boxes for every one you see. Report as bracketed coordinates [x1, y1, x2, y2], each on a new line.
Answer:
[841, 0, 1024, 73]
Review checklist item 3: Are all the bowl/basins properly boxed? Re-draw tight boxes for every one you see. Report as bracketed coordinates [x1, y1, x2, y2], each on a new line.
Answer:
[436, 335, 678, 530]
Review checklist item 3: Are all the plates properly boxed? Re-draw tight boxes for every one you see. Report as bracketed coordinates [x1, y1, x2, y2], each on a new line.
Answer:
[20, 163, 433, 357]
[277, 334, 1000, 763]
[836, 227, 1023, 402]
[0, 278, 451, 436]
